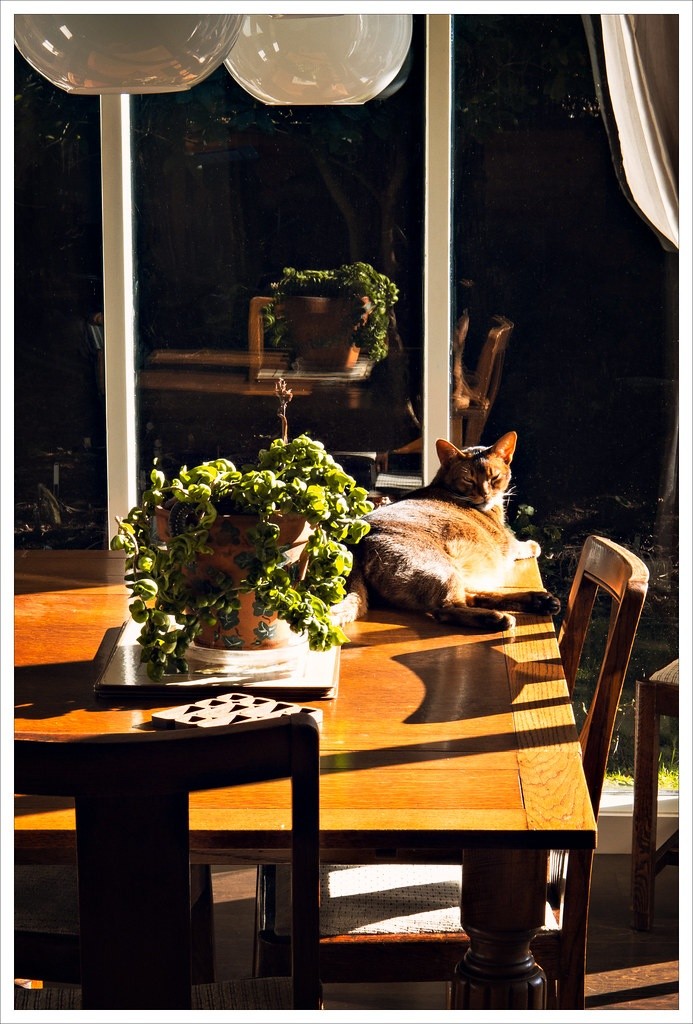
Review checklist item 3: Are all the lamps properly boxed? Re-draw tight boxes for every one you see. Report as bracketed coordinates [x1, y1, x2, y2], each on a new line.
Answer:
[14, 15, 413, 106]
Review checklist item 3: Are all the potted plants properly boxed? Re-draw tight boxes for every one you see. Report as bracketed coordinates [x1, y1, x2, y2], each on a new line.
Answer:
[107, 433, 377, 679]
[260, 261, 400, 371]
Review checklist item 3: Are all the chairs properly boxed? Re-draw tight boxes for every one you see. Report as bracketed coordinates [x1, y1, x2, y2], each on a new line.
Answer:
[14, 717, 326, 1011]
[333, 301, 516, 494]
[629, 658, 679, 929]
[253, 534, 650, 1010]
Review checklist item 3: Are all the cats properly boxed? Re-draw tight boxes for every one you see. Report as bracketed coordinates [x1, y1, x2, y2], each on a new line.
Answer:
[355, 430, 561, 630]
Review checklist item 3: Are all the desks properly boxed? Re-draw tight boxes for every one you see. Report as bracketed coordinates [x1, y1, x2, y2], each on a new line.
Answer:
[14, 549, 597, 1010]
[138, 349, 411, 474]
